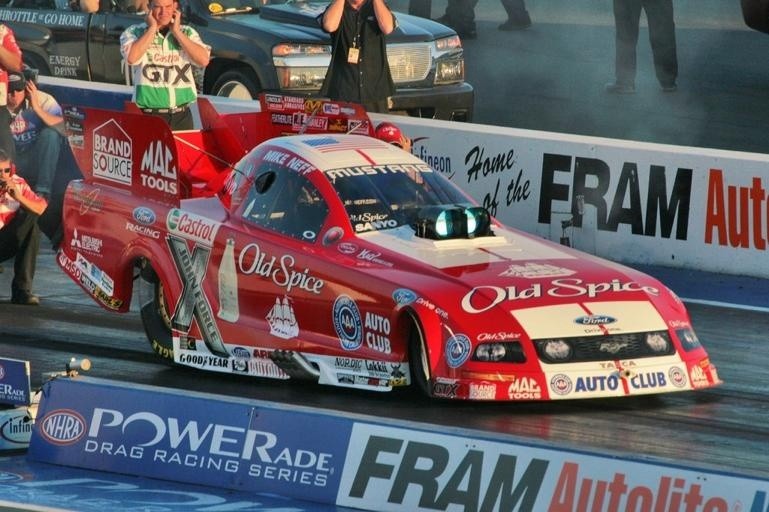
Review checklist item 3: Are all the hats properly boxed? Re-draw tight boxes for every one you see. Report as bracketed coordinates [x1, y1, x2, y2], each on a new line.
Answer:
[375, 120, 404, 149]
[6, 70, 25, 93]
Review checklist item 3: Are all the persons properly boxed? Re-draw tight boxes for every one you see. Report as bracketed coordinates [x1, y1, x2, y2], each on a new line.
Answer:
[6, 72, 69, 206]
[605, 0, 681, 95]
[430, 0, 533, 32]
[374, 121, 425, 184]
[314, 0, 399, 115]
[407, 0, 478, 40]
[78, 0, 150, 16]
[0, 20, 24, 160]
[118, 0, 213, 130]
[0, 147, 48, 306]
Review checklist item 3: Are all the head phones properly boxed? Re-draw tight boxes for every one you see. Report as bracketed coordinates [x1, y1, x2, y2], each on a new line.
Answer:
[0, 149, 16, 177]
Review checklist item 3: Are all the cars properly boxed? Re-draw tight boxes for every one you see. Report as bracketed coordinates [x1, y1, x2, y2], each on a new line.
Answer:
[0, 0, 474, 122]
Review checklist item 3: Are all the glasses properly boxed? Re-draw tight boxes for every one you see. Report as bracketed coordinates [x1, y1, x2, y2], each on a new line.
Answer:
[0, 168, 10, 174]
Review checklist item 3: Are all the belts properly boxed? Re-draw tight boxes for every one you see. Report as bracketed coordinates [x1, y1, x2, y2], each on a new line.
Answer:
[138, 103, 190, 115]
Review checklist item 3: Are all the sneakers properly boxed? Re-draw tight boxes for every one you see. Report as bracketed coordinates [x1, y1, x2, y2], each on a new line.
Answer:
[605, 81, 679, 94]
[498, 8, 534, 33]
[430, 14, 481, 41]
[10, 289, 41, 305]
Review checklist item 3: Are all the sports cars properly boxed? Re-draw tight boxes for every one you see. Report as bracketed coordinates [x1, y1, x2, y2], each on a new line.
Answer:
[56, 93, 724, 402]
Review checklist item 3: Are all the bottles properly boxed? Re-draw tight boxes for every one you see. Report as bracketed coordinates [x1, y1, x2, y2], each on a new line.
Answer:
[215, 237, 240, 325]
